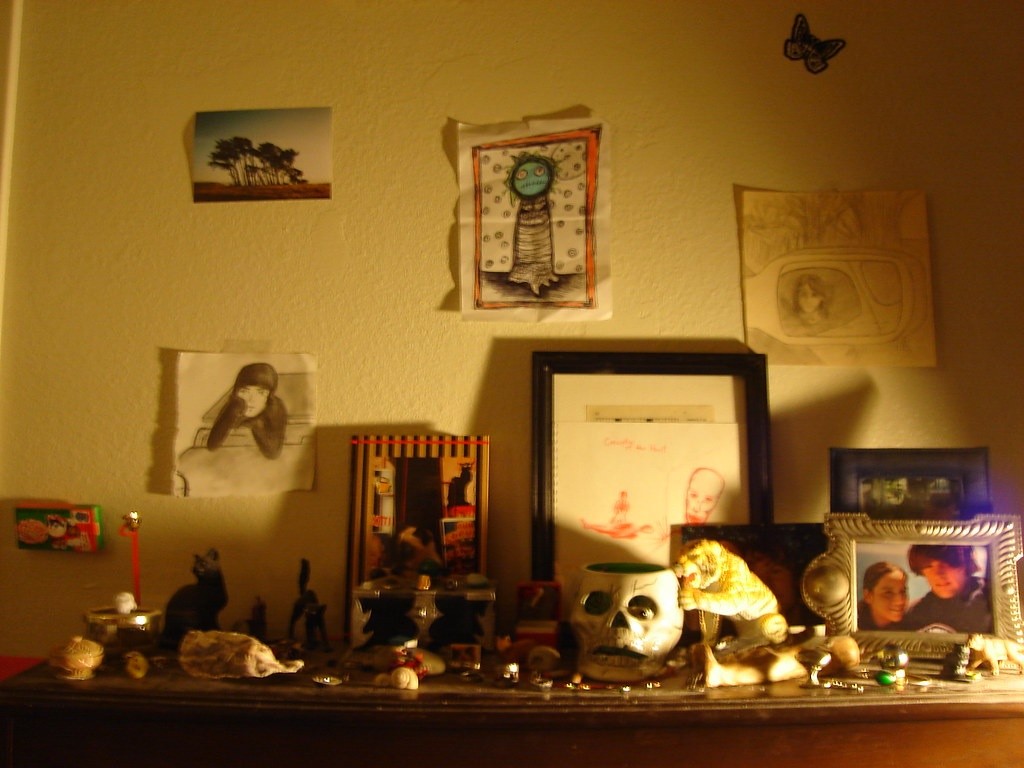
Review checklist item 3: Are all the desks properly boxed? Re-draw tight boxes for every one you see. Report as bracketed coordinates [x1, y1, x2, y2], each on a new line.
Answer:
[0, 637, 1022, 768]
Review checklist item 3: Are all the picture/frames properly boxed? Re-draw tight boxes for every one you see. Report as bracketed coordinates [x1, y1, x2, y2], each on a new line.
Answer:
[804, 510, 1023, 664]
[531, 350, 776, 585]
[826, 445, 992, 520]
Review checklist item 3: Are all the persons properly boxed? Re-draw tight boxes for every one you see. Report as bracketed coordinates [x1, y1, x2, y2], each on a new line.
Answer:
[861, 475, 954, 513]
[856, 544, 991, 635]
